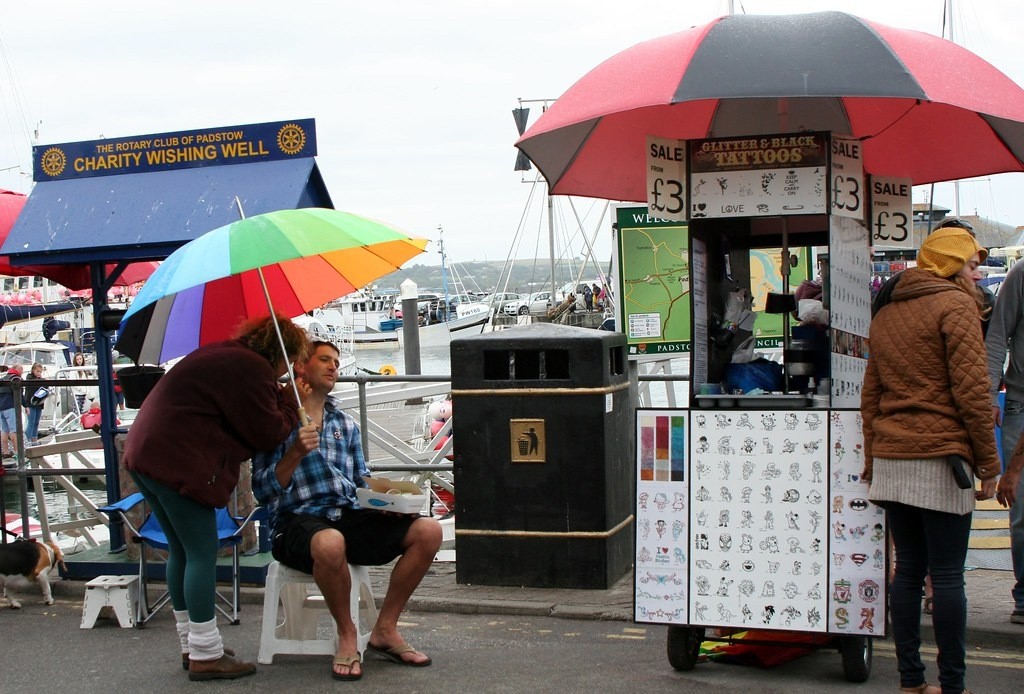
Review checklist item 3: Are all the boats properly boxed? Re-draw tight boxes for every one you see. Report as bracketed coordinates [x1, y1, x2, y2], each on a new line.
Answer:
[0, 96, 615, 492]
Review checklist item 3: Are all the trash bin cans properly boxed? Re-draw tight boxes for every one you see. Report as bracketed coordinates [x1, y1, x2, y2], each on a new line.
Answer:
[446, 321, 640, 591]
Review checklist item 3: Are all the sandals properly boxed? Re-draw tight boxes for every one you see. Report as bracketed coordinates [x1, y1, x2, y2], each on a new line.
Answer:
[901, 681, 971, 694]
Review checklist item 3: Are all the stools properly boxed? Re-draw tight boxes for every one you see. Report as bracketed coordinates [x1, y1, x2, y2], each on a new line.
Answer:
[80, 575, 144, 629]
[258, 560, 377, 664]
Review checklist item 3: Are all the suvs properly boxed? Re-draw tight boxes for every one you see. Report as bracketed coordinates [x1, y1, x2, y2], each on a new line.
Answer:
[394, 293, 441, 316]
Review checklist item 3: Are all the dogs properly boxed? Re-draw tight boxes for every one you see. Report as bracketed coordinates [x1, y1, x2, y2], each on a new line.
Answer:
[0, 540, 68, 610]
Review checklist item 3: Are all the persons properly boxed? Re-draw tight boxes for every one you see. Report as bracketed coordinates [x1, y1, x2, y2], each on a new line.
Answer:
[251, 342, 442, 682]
[67, 353, 89, 415]
[80, 402, 120, 430]
[24, 363, 48, 446]
[583, 283, 608, 313]
[121, 311, 312, 681]
[112, 361, 125, 410]
[0, 364, 30, 458]
[861, 218, 1024, 694]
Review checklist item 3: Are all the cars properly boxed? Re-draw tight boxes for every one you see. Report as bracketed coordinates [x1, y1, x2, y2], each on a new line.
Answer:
[446, 294, 483, 313]
[479, 292, 521, 315]
[504, 291, 552, 315]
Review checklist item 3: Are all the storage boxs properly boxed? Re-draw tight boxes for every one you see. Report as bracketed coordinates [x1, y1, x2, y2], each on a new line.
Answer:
[356, 474, 428, 514]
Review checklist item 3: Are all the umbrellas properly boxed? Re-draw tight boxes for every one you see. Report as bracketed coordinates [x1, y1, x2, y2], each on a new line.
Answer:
[0, 190, 161, 291]
[513, 9, 1024, 393]
[113, 194, 431, 430]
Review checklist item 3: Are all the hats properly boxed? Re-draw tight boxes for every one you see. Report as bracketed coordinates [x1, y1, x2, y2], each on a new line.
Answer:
[915, 227, 988, 278]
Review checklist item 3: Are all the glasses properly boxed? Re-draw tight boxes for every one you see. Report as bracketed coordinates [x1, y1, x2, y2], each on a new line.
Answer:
[944, 221, 974, 230]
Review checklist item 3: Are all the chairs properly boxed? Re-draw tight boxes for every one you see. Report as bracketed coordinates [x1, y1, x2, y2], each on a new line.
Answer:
[95, 492, 269, 627]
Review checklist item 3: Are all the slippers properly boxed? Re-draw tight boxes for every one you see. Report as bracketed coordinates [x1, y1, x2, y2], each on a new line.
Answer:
[367, 634, 432, 666]
[333, 646, 362, 681]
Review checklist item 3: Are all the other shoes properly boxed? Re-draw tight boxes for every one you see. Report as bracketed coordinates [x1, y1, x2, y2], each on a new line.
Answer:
[1010, 611, 1024, 624]
[182, 646, 235, 672]
[923, 597, 933, 614]
[189, 653, 257, 680]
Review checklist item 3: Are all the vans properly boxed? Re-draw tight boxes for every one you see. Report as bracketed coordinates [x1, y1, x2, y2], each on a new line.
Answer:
[546, 279, 605, 313]
